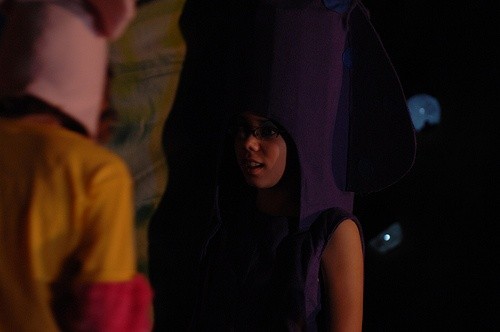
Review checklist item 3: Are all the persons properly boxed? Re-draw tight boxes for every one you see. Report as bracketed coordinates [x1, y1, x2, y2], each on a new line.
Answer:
[147, 2, 366, 332]
[0, 1, 154, 331]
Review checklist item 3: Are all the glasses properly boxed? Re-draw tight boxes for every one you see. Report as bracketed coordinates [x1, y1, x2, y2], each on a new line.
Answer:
[227, 124, 283, 144]
